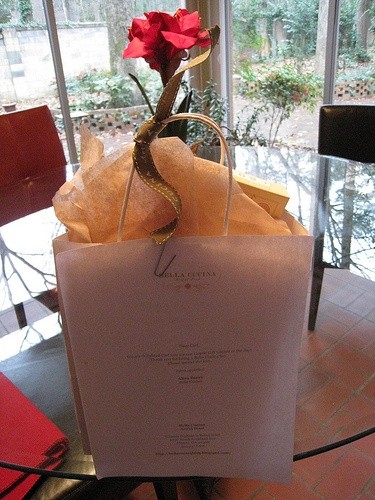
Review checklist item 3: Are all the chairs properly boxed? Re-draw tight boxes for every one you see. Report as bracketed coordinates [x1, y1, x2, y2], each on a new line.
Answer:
[0, 105, 70, 331]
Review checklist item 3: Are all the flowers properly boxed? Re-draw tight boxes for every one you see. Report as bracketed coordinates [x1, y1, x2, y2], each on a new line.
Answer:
[121, 8, 220, 145]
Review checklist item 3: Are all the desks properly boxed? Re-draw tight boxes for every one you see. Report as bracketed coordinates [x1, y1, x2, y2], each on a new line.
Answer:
[0, 140, 374, 500]
